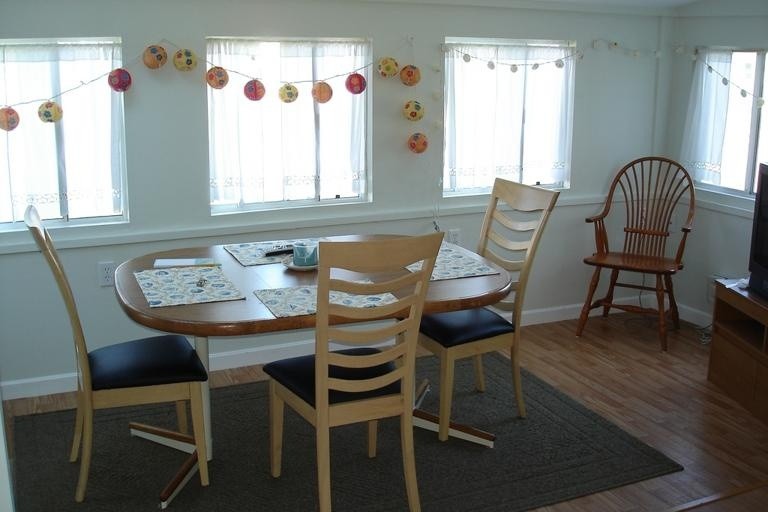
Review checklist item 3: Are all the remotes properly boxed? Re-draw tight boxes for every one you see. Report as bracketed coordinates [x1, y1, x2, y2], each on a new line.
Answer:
[261, 244, 295, 256]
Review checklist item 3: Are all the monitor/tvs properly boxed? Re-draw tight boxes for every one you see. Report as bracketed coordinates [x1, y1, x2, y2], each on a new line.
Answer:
[748, 163, 768, 302]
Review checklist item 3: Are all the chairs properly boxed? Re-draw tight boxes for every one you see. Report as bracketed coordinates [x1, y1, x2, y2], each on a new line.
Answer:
[263, 233, 444, 512]
[24, 204, 209, 503]
[396, 178, 560, 442]
[576, 157, 694, 352]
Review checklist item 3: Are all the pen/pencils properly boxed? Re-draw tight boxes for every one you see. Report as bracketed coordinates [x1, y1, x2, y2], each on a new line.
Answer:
[265, 249, 292, 255]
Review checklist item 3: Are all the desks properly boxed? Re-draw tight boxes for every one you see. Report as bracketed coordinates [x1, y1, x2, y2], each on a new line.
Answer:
[115, 234, 512, 510]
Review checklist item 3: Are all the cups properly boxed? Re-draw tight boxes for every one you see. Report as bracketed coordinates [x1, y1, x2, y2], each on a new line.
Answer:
[293, 241, 318, 266]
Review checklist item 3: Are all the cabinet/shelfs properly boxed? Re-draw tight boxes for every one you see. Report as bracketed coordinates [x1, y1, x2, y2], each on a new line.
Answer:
[706, 279, 768, 429]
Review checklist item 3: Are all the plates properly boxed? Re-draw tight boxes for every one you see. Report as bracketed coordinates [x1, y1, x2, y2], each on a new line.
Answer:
[281, 254, 319, 272]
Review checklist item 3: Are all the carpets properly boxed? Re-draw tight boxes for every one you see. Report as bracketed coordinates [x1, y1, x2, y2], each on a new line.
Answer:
[12, 351, 684, 512]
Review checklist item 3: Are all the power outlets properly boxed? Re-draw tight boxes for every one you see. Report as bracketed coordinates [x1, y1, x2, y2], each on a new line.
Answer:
[99, 262, 116, 287]
[449, 229, 461, 245]
